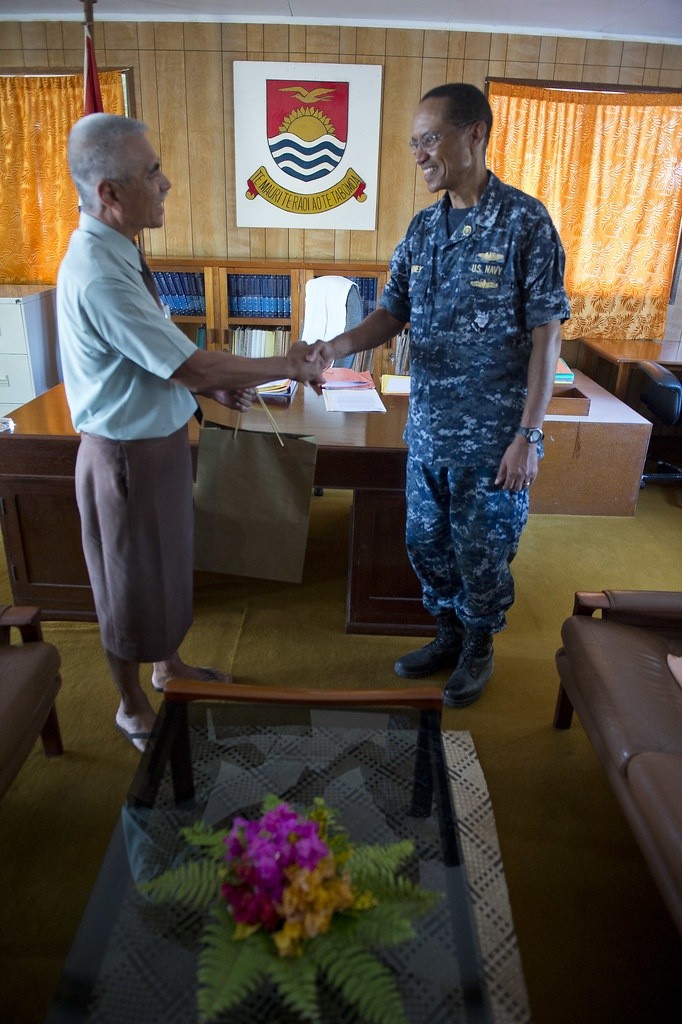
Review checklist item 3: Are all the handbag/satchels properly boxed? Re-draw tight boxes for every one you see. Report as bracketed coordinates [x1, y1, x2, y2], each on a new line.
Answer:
[190, 388, 319, 585]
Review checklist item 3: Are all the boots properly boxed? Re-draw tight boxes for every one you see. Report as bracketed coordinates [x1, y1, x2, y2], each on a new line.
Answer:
[395, 610, 465, 678]
[445, 630, 493, 707]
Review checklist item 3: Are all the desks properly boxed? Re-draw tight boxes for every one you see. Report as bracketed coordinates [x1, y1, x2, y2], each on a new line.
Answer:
[0, 374, 442, 638]
[577, 335, 682, 406]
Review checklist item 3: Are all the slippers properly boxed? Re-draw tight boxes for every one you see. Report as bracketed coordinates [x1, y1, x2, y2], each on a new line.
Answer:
[118, 724, 150, 751]
[155, 667, 232, 693]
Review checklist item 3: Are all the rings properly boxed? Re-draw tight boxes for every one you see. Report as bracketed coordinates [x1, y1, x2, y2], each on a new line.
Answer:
[524, 482, 531, 485]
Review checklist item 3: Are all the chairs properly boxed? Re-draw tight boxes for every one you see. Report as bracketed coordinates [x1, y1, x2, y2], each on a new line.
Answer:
[635, 361, 682, 489]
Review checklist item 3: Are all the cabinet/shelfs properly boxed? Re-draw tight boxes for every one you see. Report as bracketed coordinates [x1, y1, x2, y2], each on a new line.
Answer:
[0, 284, 57, 421]
[143, 258, 412, 374]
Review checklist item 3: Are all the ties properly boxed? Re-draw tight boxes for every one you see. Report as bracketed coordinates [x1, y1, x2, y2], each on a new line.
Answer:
[139, 253, 203, 425]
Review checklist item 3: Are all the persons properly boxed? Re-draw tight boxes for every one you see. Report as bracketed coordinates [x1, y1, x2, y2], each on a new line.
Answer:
[54, 114, 328, 758]
[305, 83, 571, 709]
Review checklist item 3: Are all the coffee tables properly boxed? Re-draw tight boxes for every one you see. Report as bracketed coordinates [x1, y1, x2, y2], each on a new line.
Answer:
[45, 678, 491, 1024]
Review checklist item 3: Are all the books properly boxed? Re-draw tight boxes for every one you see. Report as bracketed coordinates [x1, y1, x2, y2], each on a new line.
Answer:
[555, 357, 574, 384]
[151, 271, 410, 407]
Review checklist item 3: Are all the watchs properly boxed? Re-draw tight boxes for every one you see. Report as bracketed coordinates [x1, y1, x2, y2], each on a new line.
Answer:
[518, 427, 545, 443]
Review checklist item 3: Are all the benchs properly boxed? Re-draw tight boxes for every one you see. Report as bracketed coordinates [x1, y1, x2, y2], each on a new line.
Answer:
[552, 586, 682, 937]
[0, 605, 66, 804]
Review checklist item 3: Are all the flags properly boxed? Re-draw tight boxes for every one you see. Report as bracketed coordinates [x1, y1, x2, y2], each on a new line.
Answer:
[83, 26, 105, 118]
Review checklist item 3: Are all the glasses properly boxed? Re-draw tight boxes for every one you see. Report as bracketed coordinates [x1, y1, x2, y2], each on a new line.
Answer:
[409, 120, 478, 154]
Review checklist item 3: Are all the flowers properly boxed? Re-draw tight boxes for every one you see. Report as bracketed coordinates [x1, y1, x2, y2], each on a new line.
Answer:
[132, 791, 443, 1024]
